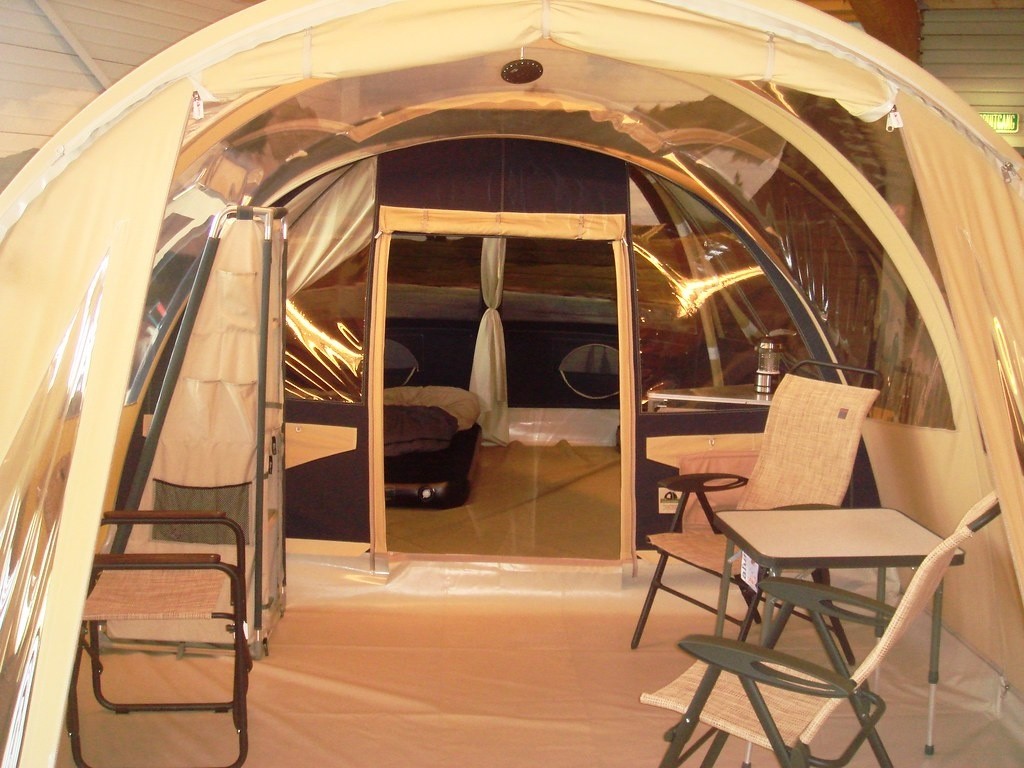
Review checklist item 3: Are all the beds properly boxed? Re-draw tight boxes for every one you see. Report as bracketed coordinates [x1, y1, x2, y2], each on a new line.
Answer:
[383, 338, 483, 510]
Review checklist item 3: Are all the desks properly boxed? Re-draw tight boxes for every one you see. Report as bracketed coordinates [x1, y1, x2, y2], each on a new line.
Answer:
[704, 507, 965, 754]
[642, 384, 769, 413]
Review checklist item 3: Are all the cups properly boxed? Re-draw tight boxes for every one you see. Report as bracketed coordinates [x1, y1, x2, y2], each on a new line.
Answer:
[754, 370, 780, 393]
[758, 340, 780, 372]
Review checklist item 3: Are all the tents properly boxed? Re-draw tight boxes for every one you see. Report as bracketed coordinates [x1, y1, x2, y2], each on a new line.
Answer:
[0, 1, 1022, 768]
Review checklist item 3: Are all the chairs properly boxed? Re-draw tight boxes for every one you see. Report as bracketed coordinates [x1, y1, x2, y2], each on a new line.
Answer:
[638, 487, 1005, 767]
[631, 357, 882, 668]
[66, 510, 251, 767]
